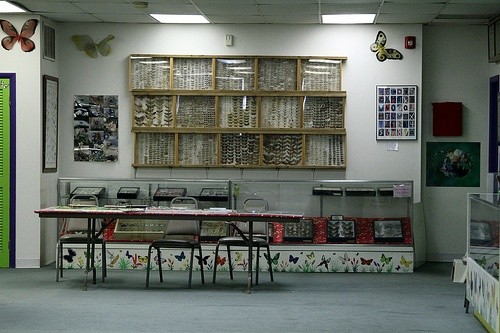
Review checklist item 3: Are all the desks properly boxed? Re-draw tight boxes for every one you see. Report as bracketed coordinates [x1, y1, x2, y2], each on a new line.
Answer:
[34, 204, 304, 295]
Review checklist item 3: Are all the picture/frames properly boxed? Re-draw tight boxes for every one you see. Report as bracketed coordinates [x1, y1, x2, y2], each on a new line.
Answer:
[43, 74, 59, 173]
[426, 142, 480, 188]
[376, 84, 417, 140]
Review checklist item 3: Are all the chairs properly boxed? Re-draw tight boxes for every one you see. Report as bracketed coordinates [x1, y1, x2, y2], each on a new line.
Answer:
[213, 198, 273, 287]
[55, 194, 107, 283]
[145, 197, 204, 289]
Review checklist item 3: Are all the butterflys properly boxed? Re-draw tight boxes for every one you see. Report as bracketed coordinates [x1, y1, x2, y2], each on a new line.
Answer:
[0, 19, 39, 52]
[370, 30, 403, 62]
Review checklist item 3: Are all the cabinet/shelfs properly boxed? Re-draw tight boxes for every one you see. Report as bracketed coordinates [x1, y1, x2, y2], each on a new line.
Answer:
[464, 192, 500, 333]
[128, 52, 346, 169]
[57, 178, 413, 274]
